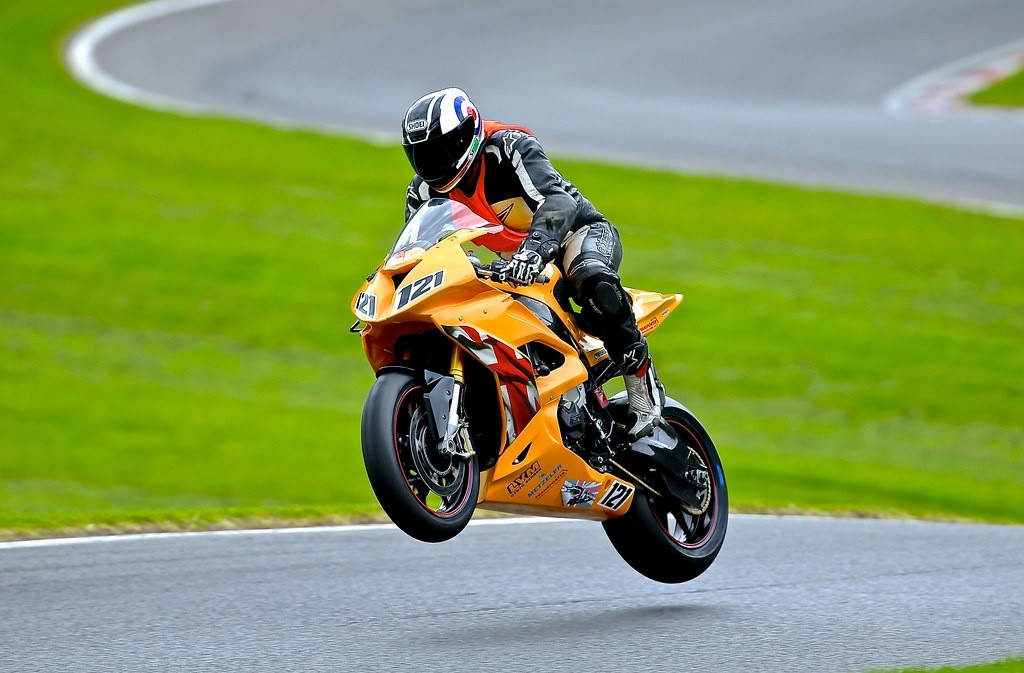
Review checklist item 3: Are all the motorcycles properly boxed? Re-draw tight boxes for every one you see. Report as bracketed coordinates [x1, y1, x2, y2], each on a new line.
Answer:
[348, 196, 730, 585]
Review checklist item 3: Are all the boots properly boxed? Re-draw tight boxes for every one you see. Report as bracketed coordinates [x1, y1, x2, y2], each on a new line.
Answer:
[616, 329, 665, 440]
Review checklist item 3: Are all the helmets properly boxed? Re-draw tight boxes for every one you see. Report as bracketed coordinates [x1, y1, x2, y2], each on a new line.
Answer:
[402, 88, 485, 193]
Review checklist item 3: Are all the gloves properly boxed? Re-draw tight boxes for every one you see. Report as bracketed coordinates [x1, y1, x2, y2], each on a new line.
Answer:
[500, 231, 561, 289]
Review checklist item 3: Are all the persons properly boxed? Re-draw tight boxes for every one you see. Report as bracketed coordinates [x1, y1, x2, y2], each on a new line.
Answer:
[400, 88, 665, 443]
